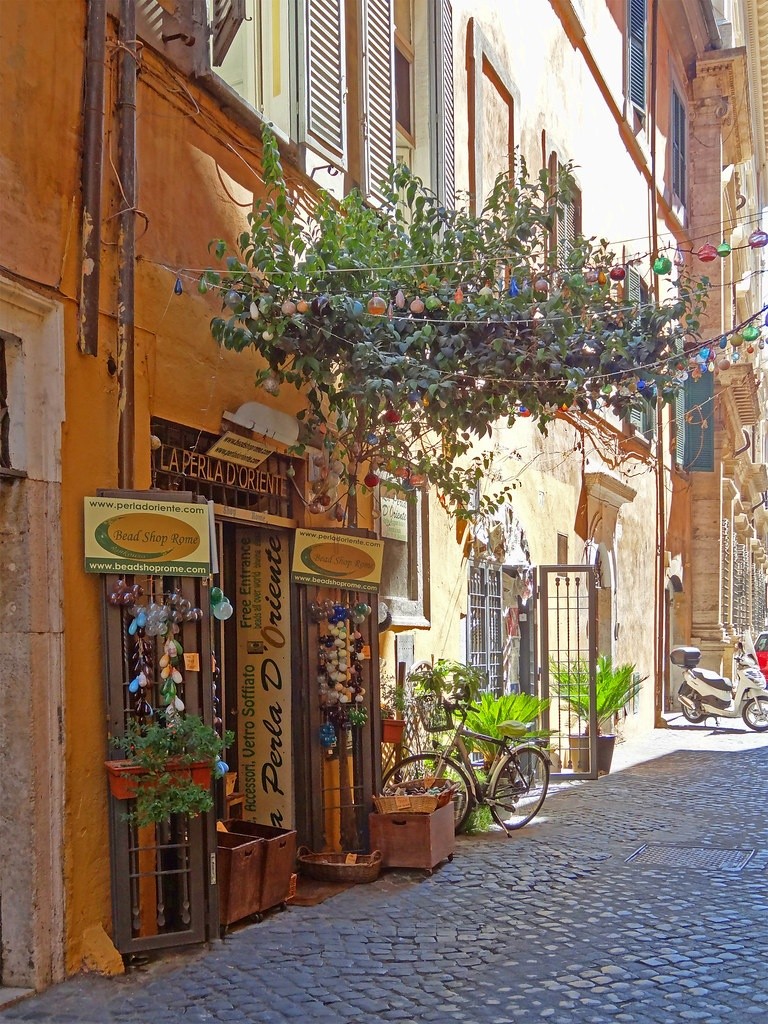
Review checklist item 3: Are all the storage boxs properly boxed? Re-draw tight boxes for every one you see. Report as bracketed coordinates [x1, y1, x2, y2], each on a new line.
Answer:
[180, 820, 298, 932]
[369, 800, 456, 876]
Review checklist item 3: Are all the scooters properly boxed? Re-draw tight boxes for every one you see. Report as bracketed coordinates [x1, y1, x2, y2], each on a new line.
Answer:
[669, 627, 768, 732]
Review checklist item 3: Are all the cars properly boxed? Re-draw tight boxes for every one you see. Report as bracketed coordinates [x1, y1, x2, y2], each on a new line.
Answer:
[754, 631, 768, 684]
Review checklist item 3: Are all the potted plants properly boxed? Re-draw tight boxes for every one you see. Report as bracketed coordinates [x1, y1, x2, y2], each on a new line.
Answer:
[105, 709, 237, 825]
[456, 692, 560, 821]
[381, 681, 411, 744]
[550, 652, 651, 776]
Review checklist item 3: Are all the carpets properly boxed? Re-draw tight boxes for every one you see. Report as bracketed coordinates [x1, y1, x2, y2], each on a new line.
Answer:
[283, 881, 354, 907]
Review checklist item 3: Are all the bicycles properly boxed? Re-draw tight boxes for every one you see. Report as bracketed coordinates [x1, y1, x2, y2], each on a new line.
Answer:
[381, 691, 554, 839]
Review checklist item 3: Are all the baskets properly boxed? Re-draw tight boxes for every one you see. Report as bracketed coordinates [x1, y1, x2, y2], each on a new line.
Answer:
[437, 784, 454, 808]
[433, 779, 447, 787]
[297, 846, 383, 883]
[371, 794, 440, 815]
[388, 776, 436, 793]
[415, 694, 455, 733]
[225, 772, 237, 795]
